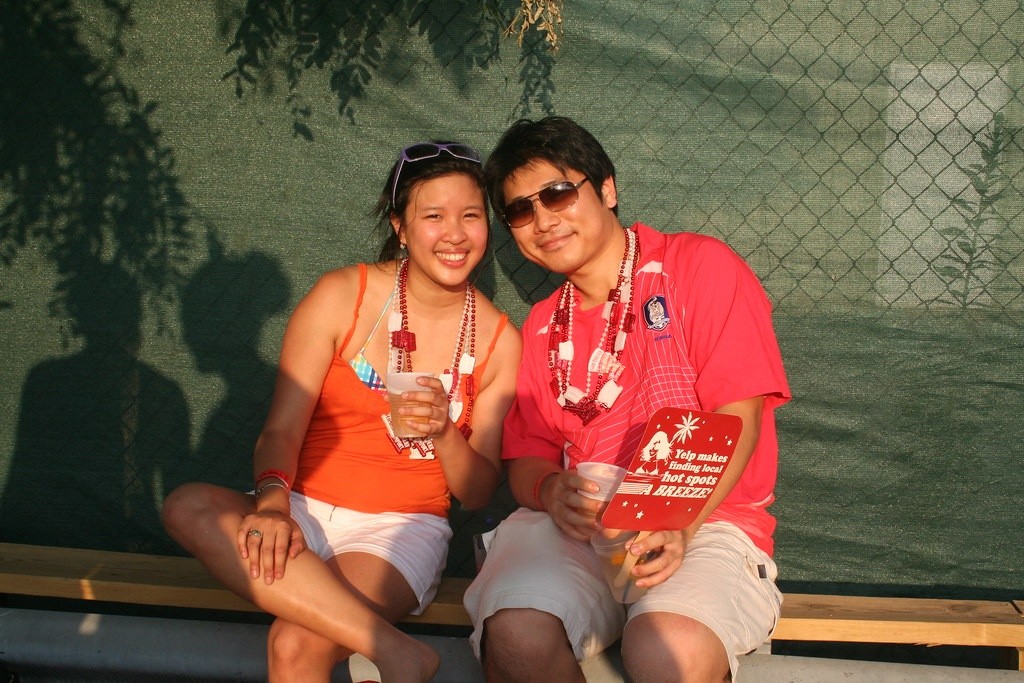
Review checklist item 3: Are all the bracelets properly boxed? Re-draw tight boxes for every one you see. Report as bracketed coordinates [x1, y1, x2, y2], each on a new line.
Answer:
[255, 468, 290, 497]
[533, 470, 557, 510]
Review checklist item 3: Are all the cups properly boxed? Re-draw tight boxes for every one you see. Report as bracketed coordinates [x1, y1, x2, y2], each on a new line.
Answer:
[575, 462, 627, 539]
[386, 372, 433, 438]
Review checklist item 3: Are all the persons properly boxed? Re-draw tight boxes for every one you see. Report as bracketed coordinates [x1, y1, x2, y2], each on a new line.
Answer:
[163, 141, 523, 683]
[461, 115, 791, 683]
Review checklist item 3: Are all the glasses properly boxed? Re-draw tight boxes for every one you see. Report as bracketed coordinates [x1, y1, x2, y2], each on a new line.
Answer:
[393, 142, 481, 209]
[500, 177, 589, 228]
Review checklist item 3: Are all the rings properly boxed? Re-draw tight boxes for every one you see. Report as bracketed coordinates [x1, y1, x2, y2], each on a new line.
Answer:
[249, 530, 262, 536]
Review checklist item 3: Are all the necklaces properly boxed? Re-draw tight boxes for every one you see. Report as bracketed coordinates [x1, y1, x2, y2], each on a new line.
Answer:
[547, 226, 639, 425]
[381, 257, 476, 459]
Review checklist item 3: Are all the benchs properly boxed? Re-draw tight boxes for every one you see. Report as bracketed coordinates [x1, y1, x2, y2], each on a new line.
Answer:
[0, 541, 1024, 683]
